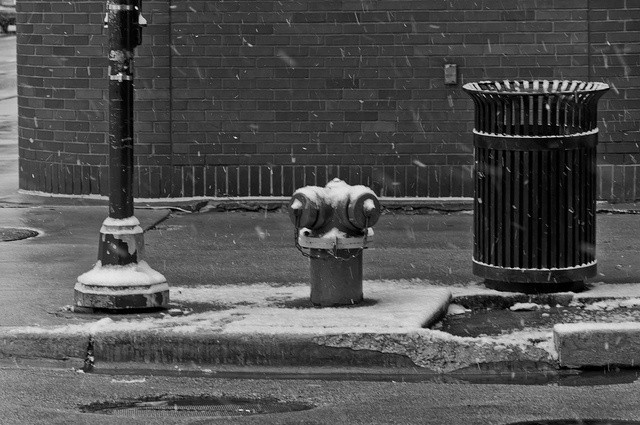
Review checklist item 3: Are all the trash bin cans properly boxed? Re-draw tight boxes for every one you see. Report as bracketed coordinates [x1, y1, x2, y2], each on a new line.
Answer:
[463, 80, 609, 291]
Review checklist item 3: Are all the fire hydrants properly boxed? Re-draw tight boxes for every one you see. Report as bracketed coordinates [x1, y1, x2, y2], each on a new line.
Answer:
[288, 178, 380, 306]
